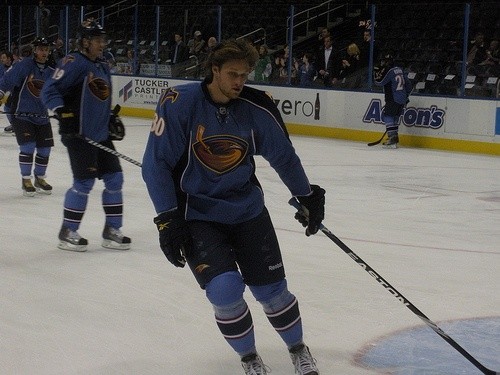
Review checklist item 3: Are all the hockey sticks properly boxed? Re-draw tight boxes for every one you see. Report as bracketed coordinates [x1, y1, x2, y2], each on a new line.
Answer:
[368, 130, 388, 146]
[0, 111, 58, 120]
[74, 134, 142, 168]
[288, 197, 500, 375]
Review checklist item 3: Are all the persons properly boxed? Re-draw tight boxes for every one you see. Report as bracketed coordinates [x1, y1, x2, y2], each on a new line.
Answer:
[40, 20, 131, 252]
[170, 31, 217, 65]
[0, 37, 74, 197]
[372, 54, 411, 149]
[468, 32, 500, 65]
[253, 28, 380, 89]
[126, 39, 326, 375]
[103, 45, 140, 74]
[38, 0, 51, 37]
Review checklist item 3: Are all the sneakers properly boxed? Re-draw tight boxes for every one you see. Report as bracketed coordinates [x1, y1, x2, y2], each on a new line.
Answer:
[382, 137, 399, 148]
[33, 175, 53, 196]
[102, 223, 130, 250]
[57, 224, 87, 253]
[4, 125, 13, 133]
[21, 177, 36, 197]
[241, 354, 271, 375]
[289, 344, 319, 375]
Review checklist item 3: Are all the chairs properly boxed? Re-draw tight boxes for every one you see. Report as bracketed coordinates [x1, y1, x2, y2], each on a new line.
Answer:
[0, 0, 499, 100]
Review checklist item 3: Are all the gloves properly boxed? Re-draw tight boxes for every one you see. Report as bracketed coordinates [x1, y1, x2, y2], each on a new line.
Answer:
[108, 104, 125, 140]
[373, 67, 381, 79]
[55, 107, 76, 138]
[154, 211, 192, 268]
[293, 185, 326, 237]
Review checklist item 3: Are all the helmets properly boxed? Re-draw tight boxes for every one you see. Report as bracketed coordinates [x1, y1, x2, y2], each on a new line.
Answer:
[382, 54, 393, 66]
[32, 36, 48, 46]
[79, 19, 106, 37]
